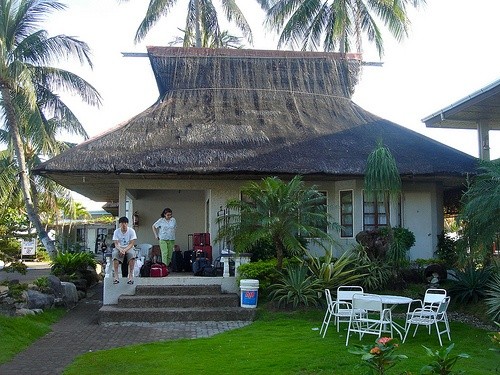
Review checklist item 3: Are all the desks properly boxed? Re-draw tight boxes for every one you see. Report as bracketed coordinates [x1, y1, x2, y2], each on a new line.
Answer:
[366, 295, 412, 340]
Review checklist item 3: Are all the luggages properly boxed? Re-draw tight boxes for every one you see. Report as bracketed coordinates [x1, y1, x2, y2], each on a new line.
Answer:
[193, 245, 212, 261]
[184, 234, 194, 272]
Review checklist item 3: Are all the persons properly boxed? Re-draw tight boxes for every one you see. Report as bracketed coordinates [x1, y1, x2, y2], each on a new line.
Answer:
[112, 216, 138, 285]
[152, 208, 176, 274]
[98, 242, 107, 253]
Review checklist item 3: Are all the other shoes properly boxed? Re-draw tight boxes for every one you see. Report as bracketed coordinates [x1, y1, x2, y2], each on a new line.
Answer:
[113, 279, 120, 284]
[127, 280, 134, 285]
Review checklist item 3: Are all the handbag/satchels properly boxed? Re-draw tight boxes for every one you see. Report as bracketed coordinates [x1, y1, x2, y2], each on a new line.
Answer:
[150, 262, 167, 276]
[140, 261, 155, 277]
[191, 250, 218, 276]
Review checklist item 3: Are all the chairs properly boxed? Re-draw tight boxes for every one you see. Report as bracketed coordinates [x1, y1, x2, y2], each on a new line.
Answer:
[318, 286, 452, 347]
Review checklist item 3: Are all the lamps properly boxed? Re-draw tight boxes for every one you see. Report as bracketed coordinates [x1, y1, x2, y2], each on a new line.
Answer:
[482, 136, 490, 150]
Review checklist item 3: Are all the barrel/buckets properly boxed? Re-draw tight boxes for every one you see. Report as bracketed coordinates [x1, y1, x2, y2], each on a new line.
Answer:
[239, 279, 259, 309]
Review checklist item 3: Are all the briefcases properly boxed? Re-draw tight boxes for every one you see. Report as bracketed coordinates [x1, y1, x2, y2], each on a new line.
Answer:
[193, 232, 210, 246]
